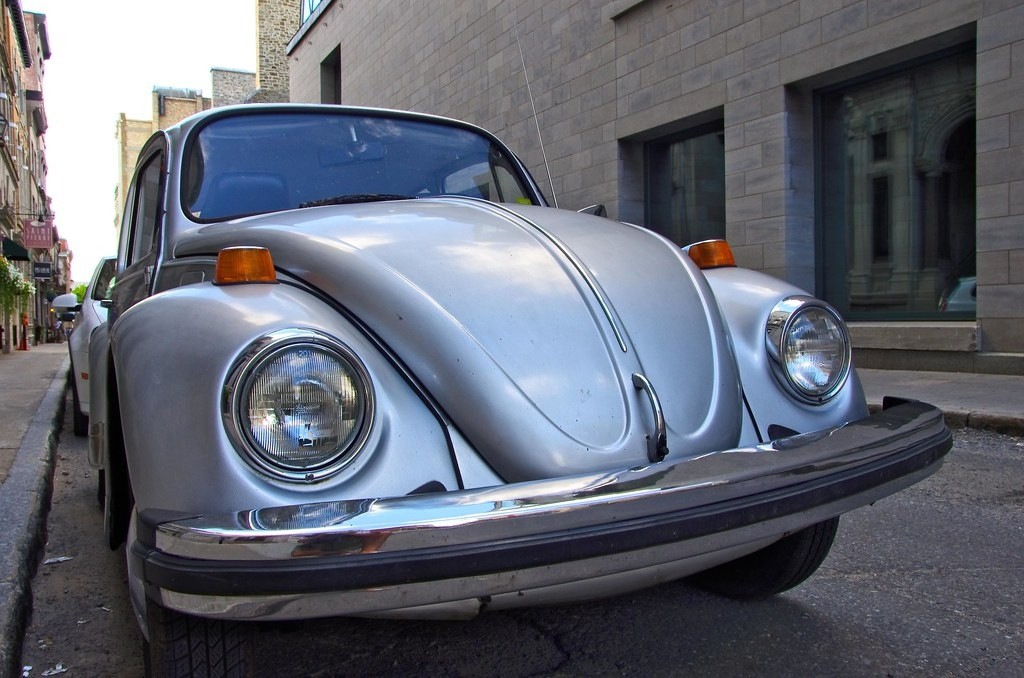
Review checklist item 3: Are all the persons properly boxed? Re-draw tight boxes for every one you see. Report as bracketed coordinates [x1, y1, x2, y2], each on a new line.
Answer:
[55, 318, 62, 340]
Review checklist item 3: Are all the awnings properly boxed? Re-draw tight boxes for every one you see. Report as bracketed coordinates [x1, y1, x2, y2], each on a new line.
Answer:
[3, 237, 32, 261]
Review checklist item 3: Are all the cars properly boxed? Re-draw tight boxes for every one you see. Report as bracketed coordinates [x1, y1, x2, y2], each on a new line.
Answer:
[50, 256, 118, 437]
[938, 276, 977, 324]
[87, 103, 954, 678]
[58, 311, 76, 337]
